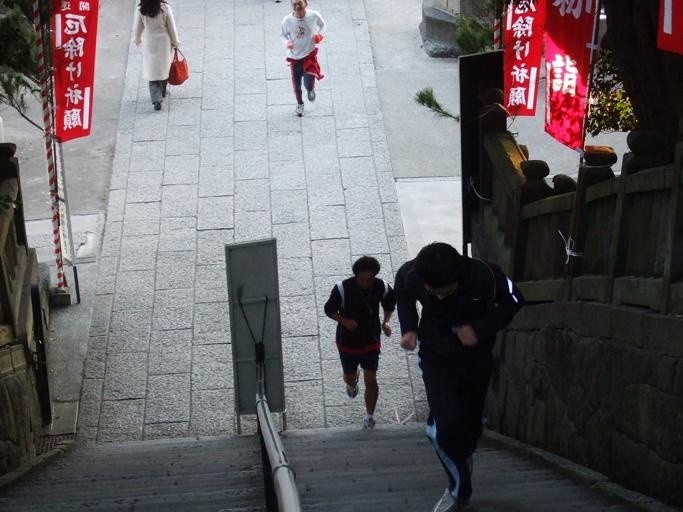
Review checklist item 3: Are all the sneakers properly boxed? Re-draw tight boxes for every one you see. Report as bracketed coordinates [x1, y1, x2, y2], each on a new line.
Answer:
[361, 416, 375, 431]
[152, 101, 162, 111]
[429, 488, 474, 512]
[306, 89, 315, 102]
[342, 367, 361, 400]
[295, 102, 304, 117]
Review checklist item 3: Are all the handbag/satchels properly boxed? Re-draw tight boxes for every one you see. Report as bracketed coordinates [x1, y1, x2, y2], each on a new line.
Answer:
[167, 46, 189, 86]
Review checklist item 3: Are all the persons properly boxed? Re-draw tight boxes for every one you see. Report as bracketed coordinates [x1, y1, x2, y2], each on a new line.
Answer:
[324, 255, 395, 430]
[394, 241, 523, 512]
[135, 0, 179, 110]
[281, 0, 326, 115]
